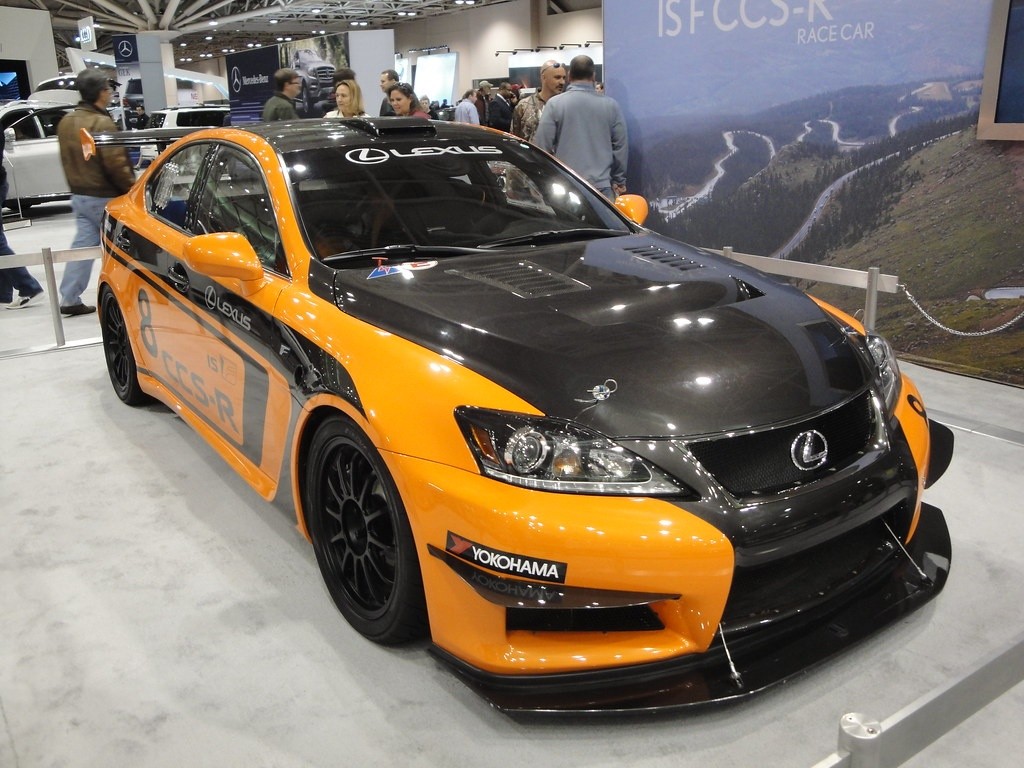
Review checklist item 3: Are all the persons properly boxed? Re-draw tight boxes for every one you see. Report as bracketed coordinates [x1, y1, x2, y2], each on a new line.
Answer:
[454, 90, 481, 125]
[534, 55, 629, 207]
[388, 82, 432, 120]
[320, 68, 356, 118]
[260, 68, 301, 122]
[511, 60, 566, 148]
[596, 81, 605, 93]
[117, 106, 148, 129]
[473, 80, 493, 126]
[320, 79, 372, 119]
[429, 99, 462, 110]
[0, 119, 47, 309]
[489, 78, 526, 132]
[418, 96, 440, 120]
[379, 69, 403, 116]
[57, 67, 140, 316]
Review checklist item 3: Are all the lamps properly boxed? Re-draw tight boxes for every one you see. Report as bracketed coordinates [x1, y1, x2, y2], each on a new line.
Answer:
[535, 45, 558, 53]
[558, 43, 581, 50]
[585, 40, 602, 49]
[495, 50, 516, 57]
[409, 45, 453, 55]
[511, 48, 534, 55]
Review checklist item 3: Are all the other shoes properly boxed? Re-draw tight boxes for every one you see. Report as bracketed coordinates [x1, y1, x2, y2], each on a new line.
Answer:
[60, 303, 97, 315]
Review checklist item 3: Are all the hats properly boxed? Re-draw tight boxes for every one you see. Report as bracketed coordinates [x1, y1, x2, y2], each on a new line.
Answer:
[512, 84, 522, 90]
[479, 81, 494, 89]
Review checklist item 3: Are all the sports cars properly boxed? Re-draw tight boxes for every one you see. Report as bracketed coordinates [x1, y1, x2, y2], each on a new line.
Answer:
[92, 128, 951, 724]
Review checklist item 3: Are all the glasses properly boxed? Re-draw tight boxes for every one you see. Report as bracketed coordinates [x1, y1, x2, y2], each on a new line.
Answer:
[394, 82, 412, 94]
[290, 80, 302, 85]
[542, 63, 565, 74]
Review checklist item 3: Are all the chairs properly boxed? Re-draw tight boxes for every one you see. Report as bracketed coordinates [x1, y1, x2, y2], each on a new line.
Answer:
[384, 178, 498, 244]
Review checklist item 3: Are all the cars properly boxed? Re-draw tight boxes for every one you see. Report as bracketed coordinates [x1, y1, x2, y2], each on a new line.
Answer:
[0, 75, 232, 213]
[292, 49, 338, 115]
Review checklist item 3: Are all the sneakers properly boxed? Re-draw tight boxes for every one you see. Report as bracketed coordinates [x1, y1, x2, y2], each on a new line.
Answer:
[5, 291, 45, 310]
[0, 297, 12, 306]
